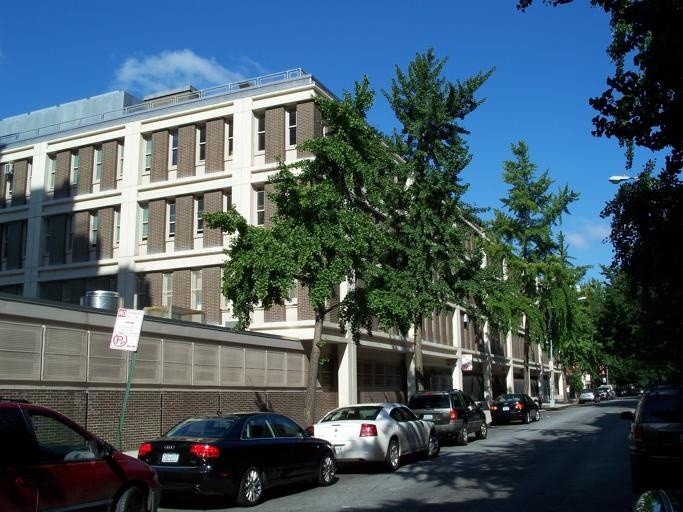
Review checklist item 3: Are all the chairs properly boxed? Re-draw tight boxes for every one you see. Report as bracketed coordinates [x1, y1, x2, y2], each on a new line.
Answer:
[347, 407, 363, 419]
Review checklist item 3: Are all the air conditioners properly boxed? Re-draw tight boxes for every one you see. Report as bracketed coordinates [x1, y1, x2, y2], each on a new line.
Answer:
[4, 164, 14, 176]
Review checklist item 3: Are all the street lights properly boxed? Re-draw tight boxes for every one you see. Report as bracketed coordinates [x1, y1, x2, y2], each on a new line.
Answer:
[608, 175, 683, 185]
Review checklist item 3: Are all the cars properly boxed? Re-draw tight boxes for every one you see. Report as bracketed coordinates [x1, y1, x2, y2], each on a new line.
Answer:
[489, 392, 541, 425]
[1, 394, 163, 512]
[298, 401, 445, 474]
[577, 383, 617, 405]
[136, 410, 339, 510]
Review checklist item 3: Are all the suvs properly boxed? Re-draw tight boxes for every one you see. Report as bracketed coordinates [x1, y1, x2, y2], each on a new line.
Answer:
[402, 388, 487, 447]
[619, 382, 683, 486]
